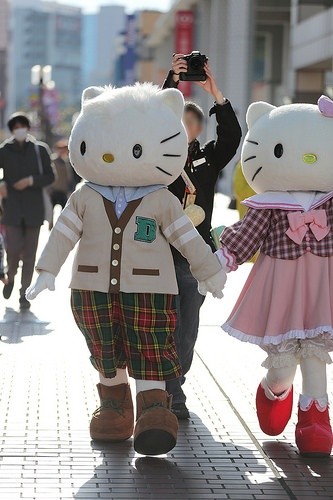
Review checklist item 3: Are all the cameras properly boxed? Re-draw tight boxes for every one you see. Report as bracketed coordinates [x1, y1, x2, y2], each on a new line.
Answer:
[179, 51, 207, 81]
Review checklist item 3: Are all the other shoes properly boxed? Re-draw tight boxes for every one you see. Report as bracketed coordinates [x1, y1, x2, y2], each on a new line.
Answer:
[19, 298, 30, 308]
[172, 403, 190, 418]
[2, 282, 14, 299]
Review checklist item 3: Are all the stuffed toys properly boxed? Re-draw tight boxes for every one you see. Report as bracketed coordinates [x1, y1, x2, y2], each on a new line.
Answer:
[26, 81, 227, 456]
[213, 95, 333, 458]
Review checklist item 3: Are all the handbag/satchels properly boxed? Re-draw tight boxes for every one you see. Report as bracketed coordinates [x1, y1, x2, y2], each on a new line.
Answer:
[43, 188, 52, 229]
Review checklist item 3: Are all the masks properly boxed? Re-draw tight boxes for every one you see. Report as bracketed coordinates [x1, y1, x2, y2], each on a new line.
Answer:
[13, 128, 28, 142]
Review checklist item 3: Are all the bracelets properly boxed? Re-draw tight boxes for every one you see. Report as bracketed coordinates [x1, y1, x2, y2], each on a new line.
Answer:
[173, 71, 179, 76]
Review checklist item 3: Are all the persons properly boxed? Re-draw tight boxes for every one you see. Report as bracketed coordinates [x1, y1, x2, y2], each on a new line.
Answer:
[162, 53, 243, 420]
[0, 110, 82, 310]
